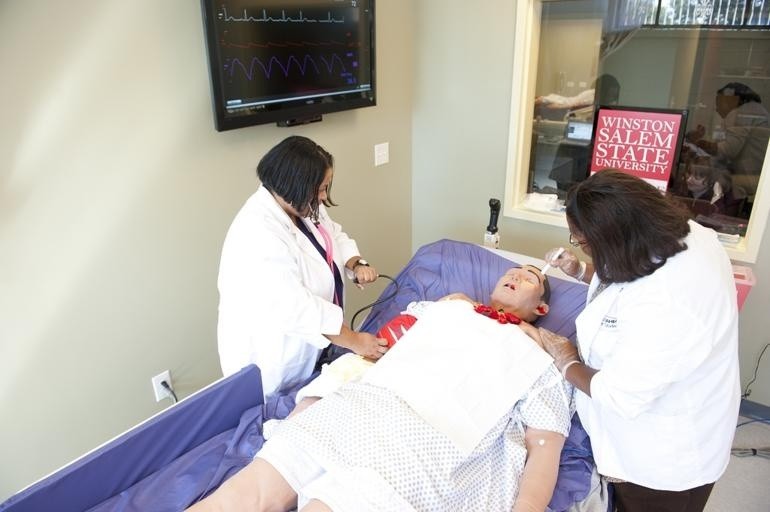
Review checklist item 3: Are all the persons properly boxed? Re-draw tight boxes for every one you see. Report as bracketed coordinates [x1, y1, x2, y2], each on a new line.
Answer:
[178, 260, 576, 512]
[590, 72, 622, 109]
[536, 167, 745, 512]
[678, 81, 770, 216]
[215, 133, 389, 413]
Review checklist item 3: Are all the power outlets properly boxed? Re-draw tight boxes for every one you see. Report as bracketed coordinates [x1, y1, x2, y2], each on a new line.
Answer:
[151, 370, 174, 402]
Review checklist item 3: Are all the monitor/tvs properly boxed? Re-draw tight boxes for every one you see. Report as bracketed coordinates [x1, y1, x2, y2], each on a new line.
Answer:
[201, 0, 376, 132]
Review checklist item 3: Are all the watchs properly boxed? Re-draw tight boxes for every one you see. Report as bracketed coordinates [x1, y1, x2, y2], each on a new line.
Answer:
[352, 258, 373, 271]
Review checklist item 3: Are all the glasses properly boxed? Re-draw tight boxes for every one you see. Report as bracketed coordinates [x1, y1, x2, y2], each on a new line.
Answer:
[568, 233, 590, 249]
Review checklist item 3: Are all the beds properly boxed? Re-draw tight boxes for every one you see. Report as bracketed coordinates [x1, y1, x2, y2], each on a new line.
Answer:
[0, 239, 609, 512]
